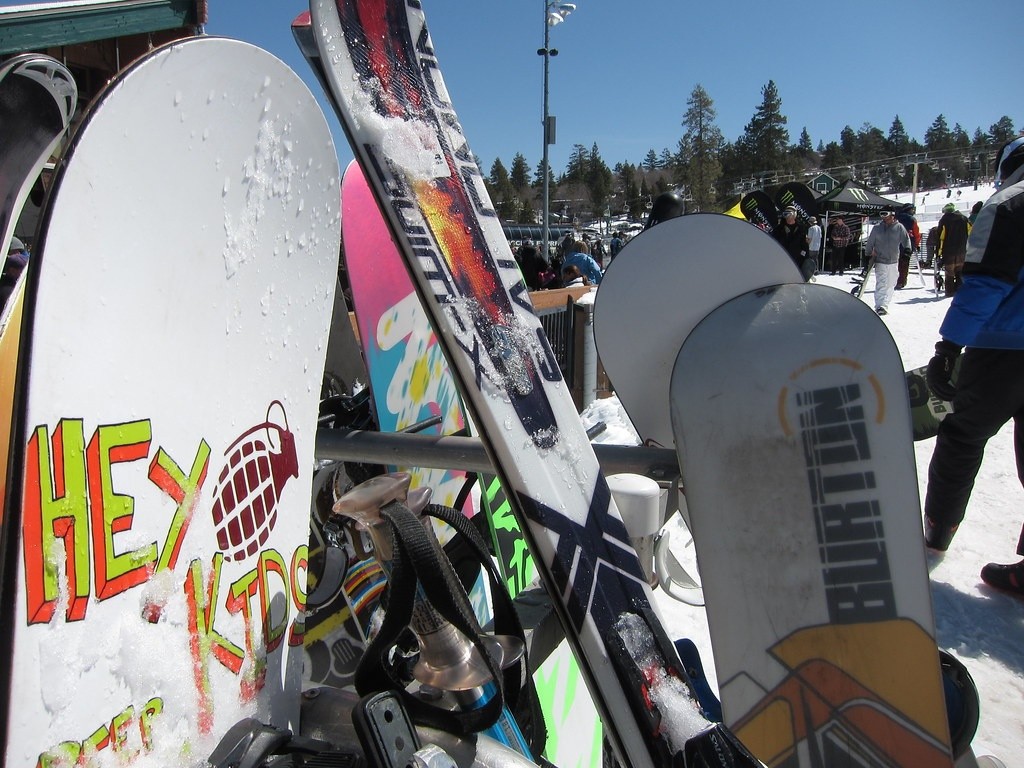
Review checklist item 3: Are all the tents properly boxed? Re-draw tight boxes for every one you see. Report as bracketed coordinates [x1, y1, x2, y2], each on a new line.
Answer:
[816, 179, 916, 272]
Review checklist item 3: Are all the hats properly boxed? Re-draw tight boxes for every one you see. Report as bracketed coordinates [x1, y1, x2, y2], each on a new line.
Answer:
[809, 217, 818, 224]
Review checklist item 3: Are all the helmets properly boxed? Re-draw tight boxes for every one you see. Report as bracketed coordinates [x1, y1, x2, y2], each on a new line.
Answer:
[903, 203, 915, 216]
[995, 136, 1024, 186]
[880, 204, 896, 217]
[942, 203, 956, 212]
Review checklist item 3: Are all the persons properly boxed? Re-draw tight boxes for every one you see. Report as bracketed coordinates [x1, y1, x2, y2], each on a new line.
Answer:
[511, 230, 632, 289]
[924, 138, 1024, 598]
[771, 190, 984, 315]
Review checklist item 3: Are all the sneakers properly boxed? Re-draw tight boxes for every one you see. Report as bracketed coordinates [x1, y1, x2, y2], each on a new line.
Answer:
[981, 558, 1024, 601]
[875, 307, 885, 315]
[922, 515, 959, 551]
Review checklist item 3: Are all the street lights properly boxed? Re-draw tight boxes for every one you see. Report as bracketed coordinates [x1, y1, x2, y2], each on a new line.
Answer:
[537, 0, 578, 263]
